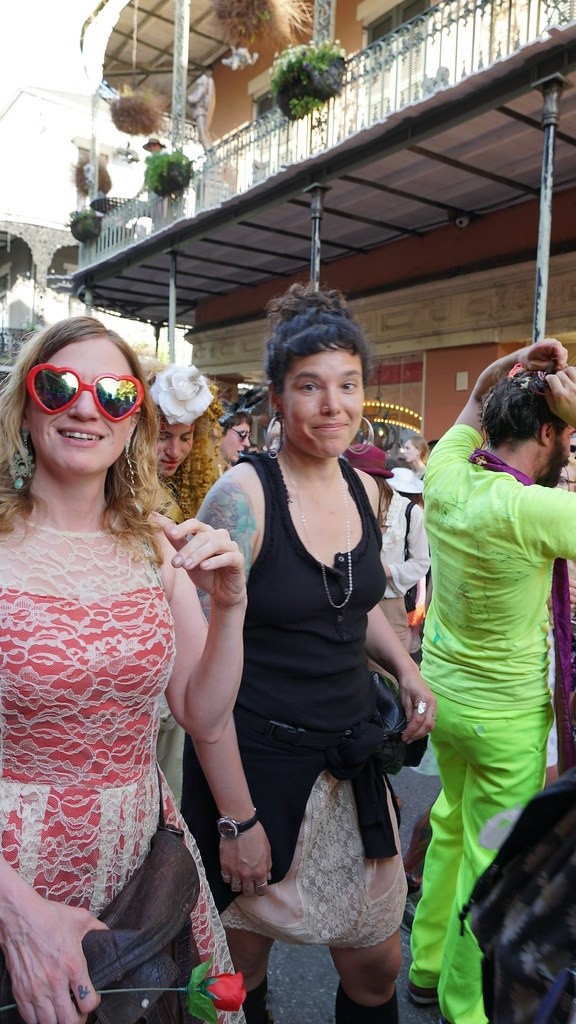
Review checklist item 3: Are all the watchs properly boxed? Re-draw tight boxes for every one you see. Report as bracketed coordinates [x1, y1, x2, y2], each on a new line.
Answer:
[216, 807, 258, 839]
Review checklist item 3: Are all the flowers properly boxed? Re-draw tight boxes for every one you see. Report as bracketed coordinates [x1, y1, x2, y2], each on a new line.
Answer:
[0, 961, 245, 1018]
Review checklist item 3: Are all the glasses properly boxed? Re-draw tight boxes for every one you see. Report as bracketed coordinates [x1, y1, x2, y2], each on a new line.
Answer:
[224, 424, 250, 439]
[25, 363, 144, 422]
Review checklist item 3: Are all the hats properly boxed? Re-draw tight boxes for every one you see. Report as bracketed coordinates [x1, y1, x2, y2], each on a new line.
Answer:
[342, 444, 394, 479]
[385, 467, 424, 493]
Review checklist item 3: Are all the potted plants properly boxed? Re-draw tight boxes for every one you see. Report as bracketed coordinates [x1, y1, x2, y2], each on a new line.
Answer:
[144, 151, 191, 195]
[271, 41, 348, 122]
[67, 205, 100, 240]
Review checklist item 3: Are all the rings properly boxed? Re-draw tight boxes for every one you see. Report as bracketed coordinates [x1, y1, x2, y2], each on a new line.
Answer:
[221, 871, 230, 879]
[415, 700, 427, 714]
[256, 882, 267, 887]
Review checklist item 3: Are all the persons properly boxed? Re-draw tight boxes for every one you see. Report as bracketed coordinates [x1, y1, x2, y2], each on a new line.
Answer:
[222, 47, 258, 71]
[140, 365, 576, 930]
[405, 336, 576, 1024]
[116, 142, 140, 164]
[186, 74, 216, 157]
[0, 313, 249, 1024]
[136, 138, 166, 197]
[178, 278, 437, 1024]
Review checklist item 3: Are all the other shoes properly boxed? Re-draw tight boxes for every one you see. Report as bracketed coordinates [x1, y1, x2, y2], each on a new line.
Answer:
[396, 873, 423, 932]
[405, 980, 440, 1004]
[438, 1016, 451, 1024]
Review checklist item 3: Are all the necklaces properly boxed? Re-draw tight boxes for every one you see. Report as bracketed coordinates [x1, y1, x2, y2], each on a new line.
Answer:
[278, 451, 354, 609]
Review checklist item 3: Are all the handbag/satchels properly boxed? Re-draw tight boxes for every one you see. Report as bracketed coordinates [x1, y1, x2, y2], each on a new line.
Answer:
[68, 822, 206, 1024]
[455, 765, 576, 1024]
[234, 670, 427, 768]
